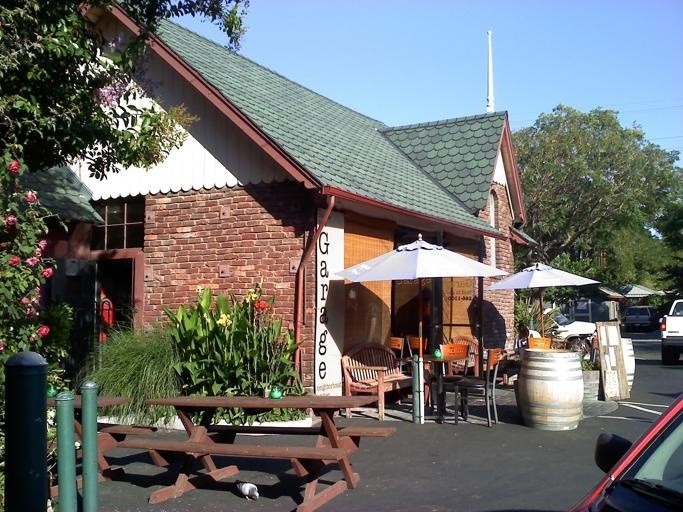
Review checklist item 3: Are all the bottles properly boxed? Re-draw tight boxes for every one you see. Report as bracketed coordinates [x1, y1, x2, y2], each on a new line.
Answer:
[47, 384, 58, 398]
[264, 386, 282, 399]
[434, 349, 441, 358]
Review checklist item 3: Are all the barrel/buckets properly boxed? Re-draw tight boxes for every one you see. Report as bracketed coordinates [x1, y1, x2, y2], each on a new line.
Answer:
[518, 349, 585, 432]
[621, 337, 636, 392]
[582, 370, 599, 401]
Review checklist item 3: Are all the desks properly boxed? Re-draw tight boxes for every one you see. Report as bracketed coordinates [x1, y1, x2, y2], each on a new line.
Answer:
[46, 396, 128, 499]
[142, 395, 378, 512]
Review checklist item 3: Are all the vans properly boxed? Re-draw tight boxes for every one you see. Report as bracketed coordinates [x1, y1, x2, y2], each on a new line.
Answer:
[624, 305, 656, 333]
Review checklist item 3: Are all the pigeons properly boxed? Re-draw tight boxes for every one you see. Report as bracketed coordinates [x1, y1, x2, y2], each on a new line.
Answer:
[234, 479, 260, 502]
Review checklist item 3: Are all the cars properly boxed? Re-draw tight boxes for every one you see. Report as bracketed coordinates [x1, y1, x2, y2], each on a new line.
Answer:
[525, 305, 597, 361]
[565, 390, 683, 511]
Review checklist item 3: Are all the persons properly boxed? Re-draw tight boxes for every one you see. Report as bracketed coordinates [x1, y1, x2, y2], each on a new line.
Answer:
[512, 322, 542, 355]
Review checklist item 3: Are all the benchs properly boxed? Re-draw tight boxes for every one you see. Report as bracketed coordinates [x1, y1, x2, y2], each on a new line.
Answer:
[115, 436, 347, 511]
[98, 423, 156, 437]
[200, 423, 393, 456]
[341, 341, 414, 423]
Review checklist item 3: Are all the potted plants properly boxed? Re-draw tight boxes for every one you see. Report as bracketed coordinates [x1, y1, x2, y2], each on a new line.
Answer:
[581, 360, 600, 402]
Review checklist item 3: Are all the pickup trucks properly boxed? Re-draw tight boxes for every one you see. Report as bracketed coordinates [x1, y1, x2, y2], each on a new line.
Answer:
[658, 299, 682, 365]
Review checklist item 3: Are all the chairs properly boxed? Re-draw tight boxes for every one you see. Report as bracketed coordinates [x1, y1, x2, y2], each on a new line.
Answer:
[392, 335, 503, 429]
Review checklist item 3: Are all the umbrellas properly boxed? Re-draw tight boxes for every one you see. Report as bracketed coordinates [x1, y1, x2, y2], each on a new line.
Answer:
[480, 258, 604, 342]
[330, 232, 511, 359]
